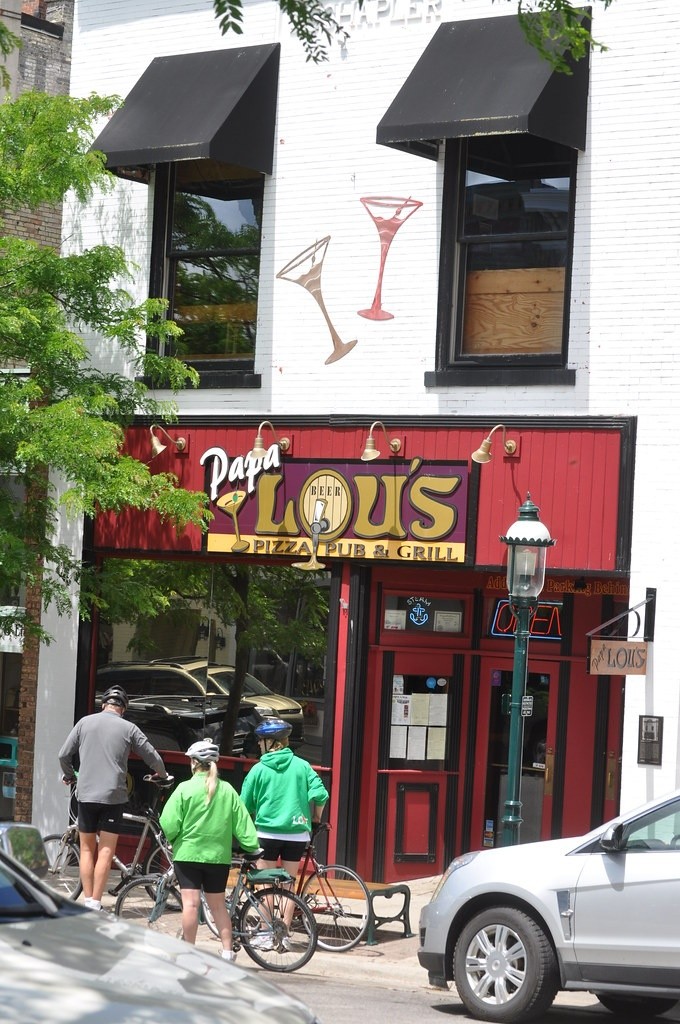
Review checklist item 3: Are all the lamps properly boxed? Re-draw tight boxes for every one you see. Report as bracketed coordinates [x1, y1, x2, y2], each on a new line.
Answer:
[149, 425, 186, 458]
[361, 421, 402, 461]
[250, 421, 290, 458]
[471, 424, 517, 464]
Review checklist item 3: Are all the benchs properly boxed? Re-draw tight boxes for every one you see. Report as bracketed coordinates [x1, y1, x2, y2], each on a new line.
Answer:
[222, 867, 412, 946]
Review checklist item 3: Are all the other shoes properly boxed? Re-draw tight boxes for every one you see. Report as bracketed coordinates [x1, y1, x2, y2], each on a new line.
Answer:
[99, 905, 111, 913]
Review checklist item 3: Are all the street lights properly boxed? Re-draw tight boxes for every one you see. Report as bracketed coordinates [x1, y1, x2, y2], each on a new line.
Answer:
[495, 491, 558, 848]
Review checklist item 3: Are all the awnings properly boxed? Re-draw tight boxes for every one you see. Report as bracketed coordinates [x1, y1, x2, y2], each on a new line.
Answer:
[86, 43, 282, 185]
[375, 7, 591, 162]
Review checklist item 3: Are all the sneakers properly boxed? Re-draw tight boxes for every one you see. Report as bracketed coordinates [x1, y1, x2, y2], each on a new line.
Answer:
[250, 935, 293, 951]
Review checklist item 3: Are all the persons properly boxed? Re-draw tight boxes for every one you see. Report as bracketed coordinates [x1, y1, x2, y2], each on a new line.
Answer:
[159, 740, 263, 961]
[59, 684, 168, 915]
[238, 720, 329, 951]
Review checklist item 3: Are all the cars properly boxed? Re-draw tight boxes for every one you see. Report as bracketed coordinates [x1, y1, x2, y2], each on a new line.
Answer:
[0, 844, 331, 1024]
[417, 787, 680, 1023]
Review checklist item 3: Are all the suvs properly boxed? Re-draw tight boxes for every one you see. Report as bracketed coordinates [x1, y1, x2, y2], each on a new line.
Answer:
[251, 648, 306, 697]
[92, 691, 267, 759]
[96, 655, 308, 754]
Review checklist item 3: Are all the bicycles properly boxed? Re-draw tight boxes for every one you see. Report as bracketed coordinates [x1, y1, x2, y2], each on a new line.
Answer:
[199, 818, 373, 953]
[41, 774, 184, 911]
[115, 805, 318, 973]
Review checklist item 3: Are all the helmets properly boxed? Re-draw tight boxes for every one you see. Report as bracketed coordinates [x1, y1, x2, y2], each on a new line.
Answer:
[254, 720, 293, 739]
[102, 685, 128, 710]
[185, 741, 219, 762]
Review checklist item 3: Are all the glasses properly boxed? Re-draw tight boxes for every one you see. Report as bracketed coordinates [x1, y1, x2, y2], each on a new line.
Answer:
[255, 736, 269, 742]
